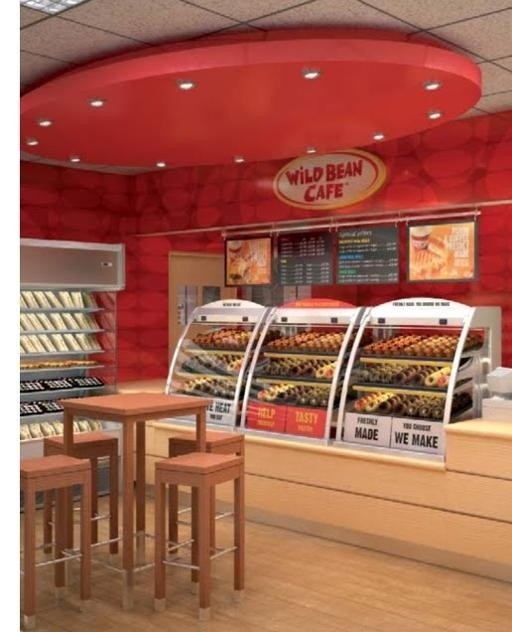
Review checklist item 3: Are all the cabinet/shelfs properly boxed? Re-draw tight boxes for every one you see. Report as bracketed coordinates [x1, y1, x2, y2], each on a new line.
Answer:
[240, 298, 373, 447]
[332, 296, 502, 463]
[163, 296, 273, 426]
[20, 286, 123, 515]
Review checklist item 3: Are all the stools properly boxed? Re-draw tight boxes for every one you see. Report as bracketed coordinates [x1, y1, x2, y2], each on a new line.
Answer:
[20, 454, 91, 625]
[168, 430, 247, 562]
[43, 431, 118, 565]
[155, 451, 245, 621]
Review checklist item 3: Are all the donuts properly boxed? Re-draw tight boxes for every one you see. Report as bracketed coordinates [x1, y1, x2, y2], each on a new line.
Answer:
[257, 330, 354, 408]
[176, 328, 278, 399]
[353, 333, 482, 419]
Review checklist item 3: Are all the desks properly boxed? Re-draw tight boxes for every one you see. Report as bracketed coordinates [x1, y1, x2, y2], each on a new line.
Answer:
[53, 391, 214, 601]
